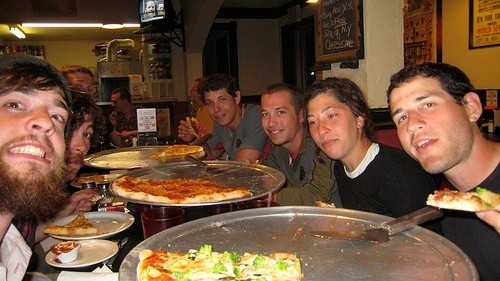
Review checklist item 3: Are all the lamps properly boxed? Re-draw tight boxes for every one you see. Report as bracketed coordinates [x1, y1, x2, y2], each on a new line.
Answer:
[9, 25, 27, 40]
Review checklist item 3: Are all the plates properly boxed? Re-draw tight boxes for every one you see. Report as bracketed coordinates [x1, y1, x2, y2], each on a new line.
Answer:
[84, 145, 206, 169]
[70, 174, 122, 189]
[109, 161, 286, 207]
[48, 211, 135, 240]
[140, 207, 186, 239]
[44, 239, 119, 268]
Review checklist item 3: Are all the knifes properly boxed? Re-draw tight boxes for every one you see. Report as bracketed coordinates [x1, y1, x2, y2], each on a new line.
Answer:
[106, 237, 129, 269]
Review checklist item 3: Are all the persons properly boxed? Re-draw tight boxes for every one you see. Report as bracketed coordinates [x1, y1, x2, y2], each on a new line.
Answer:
[59, 65, 95, 94]
[144, 0, 155, 17]
[0, 51, 75, 281]
[11, 92, 100, 251]
[177, 74, 270, 163]
[190, 78, 213, 134]
[305, 77, 442, 236]
[156, 3, 165, 15]
[244, 82, 343, 208]
[111, 88, 139, 138]
[387, 62, 500, 281]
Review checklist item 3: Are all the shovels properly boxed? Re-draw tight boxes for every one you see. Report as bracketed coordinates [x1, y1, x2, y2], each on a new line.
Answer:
[184, 154, 243, 177]
[310, 205, 444, 243]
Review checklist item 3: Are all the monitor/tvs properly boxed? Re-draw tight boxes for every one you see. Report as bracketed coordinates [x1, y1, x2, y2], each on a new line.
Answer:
[138, 0, 176, 27]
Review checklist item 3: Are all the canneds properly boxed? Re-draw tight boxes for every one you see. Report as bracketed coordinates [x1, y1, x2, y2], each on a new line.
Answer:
[26, 45, 30, 54]
[22, 44, 26, 55]
[16, 45, 22, 54]
[8, 44, 17, 54]
[0, 45, 4, 55]
[34, 46, 40, 55]
[31, 45, 33, 54]
[4, 44, 10, 56]
[41, 46, 44, 56]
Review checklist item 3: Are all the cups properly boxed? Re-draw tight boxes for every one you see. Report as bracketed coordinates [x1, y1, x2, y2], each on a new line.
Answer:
[119, 206, 478, 281]
[51, 242, 80, 263]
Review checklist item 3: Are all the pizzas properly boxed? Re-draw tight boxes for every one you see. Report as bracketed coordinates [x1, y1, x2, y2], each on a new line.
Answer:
[42, 210, 96, 236]
[135, 245, 306, 281]
[110, 175, 254, 206]
[75, 174, 106, 186]
[426, 189, 497, 213]
[146, 141, 203, 157]
[185, 116, 209, 138]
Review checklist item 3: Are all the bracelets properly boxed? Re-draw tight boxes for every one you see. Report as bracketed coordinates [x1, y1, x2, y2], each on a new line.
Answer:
[188, 136, 196, 143]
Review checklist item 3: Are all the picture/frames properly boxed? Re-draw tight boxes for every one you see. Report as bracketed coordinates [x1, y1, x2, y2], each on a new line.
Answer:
[403, 0, 442, 67]
[468, 0, 500, 50]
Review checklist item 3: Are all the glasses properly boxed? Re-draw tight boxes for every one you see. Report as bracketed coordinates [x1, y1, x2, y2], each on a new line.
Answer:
[190, 90, 198, 93]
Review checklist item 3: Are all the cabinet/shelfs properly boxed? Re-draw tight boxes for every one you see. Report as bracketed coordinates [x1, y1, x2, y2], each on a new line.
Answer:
[133, 8, 186, 52]
